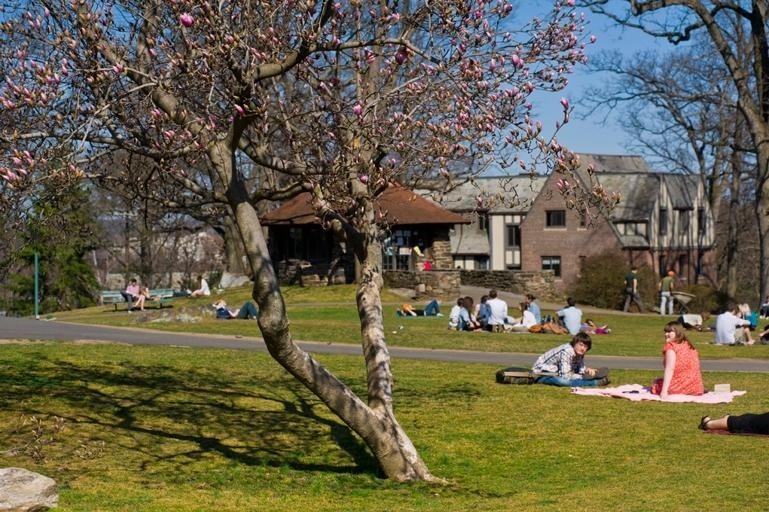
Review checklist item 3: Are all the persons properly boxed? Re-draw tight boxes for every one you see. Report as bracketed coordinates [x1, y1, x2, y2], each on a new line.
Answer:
[659, 271, 676, 315]
[624, 268, 644, 312]
[698, 411, 769, 435]
[402, 300, 443, 318]
[191, 275, 210, 297]
[678, 297, 769, 346]
[213, 299, 257, 320]
[531, 332, 610, 387]
[449, 290, 611, 335]
[125, 279, 165, 312]
[648, 324, 704, 398]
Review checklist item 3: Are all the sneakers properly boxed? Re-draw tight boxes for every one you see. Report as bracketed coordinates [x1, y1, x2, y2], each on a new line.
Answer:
[437, 314, 444, 317]
[698, 416, 710, 431]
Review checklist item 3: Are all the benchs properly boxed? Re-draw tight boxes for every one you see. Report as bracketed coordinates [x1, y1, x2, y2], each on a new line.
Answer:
[102, 288, 176, 310]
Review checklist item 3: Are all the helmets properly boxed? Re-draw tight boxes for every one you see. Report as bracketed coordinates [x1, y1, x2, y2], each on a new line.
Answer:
[668, 271, 676, 276]
